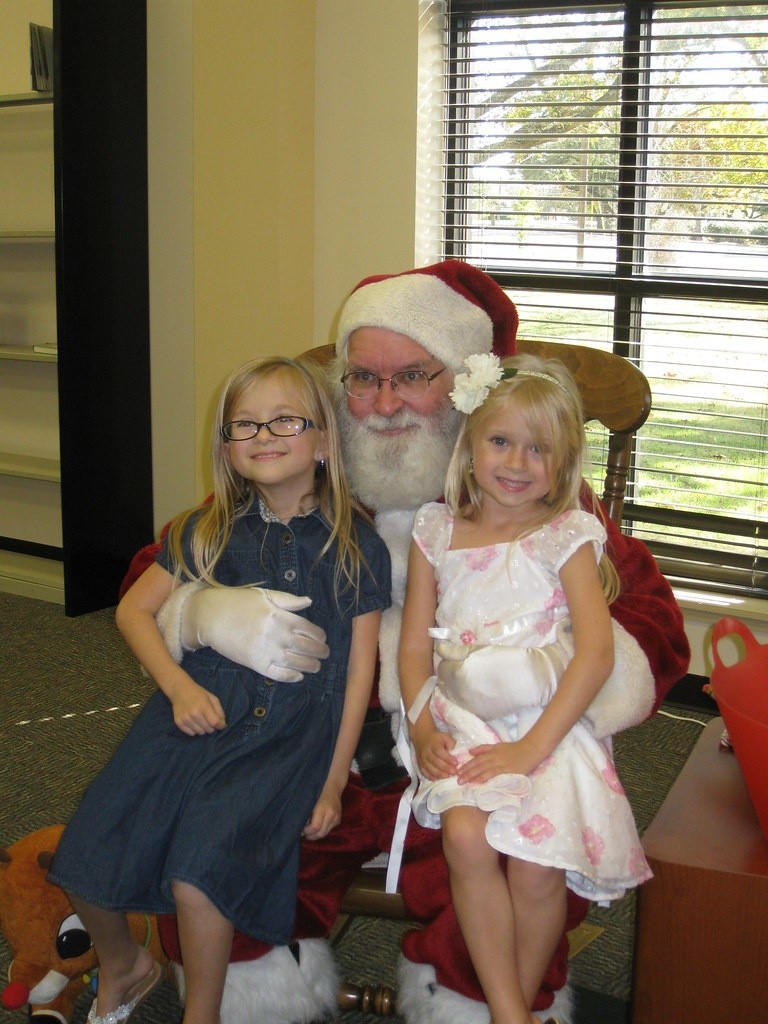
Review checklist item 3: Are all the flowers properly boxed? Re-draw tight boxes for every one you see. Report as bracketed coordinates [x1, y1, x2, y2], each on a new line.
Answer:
[447, 351, 506, 416]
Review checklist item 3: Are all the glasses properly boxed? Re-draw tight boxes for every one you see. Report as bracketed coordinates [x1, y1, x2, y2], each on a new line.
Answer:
[340, 366, 448, 398]
[222, 414, 329, 441]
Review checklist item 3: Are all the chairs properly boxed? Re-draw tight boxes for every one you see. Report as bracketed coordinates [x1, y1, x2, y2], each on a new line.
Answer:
[291, 335, 653, 1015]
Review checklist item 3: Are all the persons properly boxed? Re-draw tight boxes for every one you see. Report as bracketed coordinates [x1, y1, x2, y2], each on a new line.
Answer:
[121, 262, 690, 1022]
[398, 356, 652, 1024]
[46, 354, 392, 1024]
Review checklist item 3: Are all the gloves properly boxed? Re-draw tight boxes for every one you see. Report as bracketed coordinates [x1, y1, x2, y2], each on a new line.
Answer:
[180, 586, 332, 684]
[435, 631, 576, 723]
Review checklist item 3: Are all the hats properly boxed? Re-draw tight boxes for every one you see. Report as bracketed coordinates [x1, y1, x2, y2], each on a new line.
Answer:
[333, 259, 520, 380]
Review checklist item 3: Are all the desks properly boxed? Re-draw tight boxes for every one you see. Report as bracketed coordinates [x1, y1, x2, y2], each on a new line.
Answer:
[633, 714, 767, 1022]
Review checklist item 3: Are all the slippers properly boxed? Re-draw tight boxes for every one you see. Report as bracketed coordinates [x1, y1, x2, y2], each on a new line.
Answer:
[87, 960, 162, 1024]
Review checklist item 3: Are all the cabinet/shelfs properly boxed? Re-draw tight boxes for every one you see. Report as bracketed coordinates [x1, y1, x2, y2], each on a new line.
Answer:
[2, 90, 76, 611]
